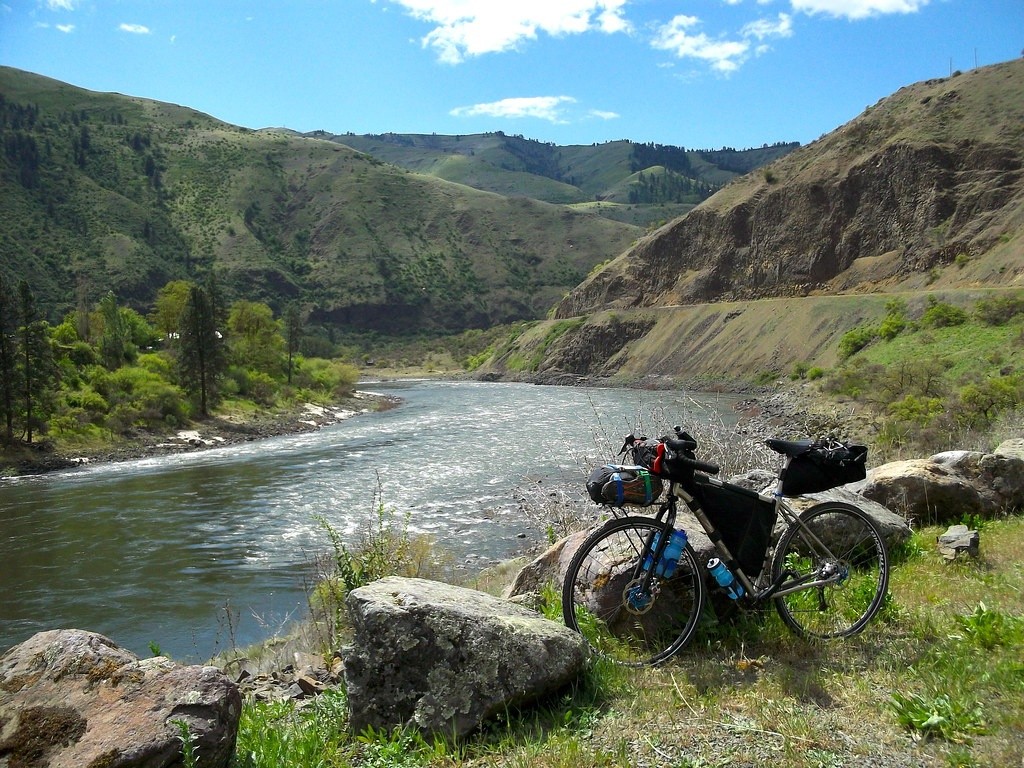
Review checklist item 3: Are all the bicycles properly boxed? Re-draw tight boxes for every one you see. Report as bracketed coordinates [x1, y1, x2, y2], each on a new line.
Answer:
[561, 425, 889, 671]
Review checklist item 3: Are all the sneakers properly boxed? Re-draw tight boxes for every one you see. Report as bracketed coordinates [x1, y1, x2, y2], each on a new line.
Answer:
[600, 474, 663, 504]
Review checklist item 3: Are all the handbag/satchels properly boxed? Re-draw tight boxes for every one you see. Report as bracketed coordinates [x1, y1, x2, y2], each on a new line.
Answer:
[783, 438, 867, 495]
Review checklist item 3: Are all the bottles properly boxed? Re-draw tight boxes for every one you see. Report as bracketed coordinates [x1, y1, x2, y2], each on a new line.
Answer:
[641, 529, 661, 571]
[707, 558, 744, 599]
[655, 529, 688, 578]
